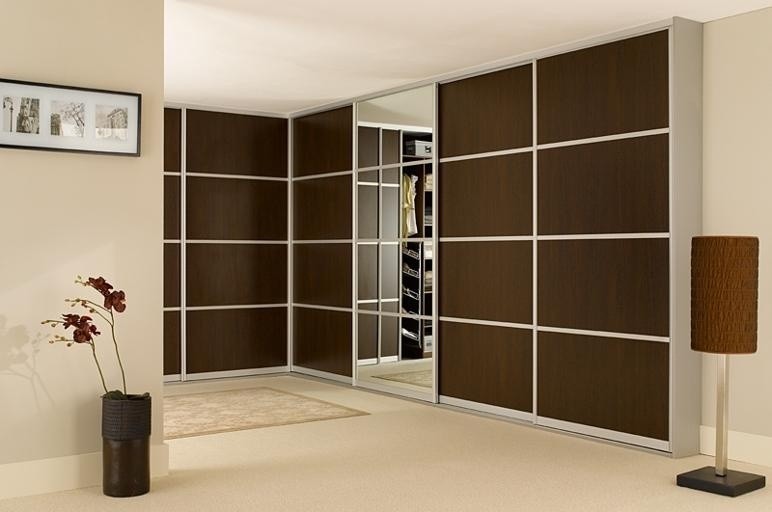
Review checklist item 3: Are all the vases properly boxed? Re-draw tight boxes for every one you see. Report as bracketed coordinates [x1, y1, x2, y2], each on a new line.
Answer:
[102, 394, 151, 498]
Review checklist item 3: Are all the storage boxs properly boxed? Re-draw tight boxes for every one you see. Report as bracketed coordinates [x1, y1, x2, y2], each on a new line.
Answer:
[406, 140, 432, 156]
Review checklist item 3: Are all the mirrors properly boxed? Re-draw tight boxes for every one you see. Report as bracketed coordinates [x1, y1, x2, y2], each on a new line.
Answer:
[352, 81, 439, 404]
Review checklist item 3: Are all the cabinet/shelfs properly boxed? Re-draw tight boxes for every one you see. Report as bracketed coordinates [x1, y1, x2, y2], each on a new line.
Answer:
[403, 155, 432, 358]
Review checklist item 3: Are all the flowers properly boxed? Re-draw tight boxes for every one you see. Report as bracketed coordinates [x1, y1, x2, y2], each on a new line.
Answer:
[40, 275, 150, 400]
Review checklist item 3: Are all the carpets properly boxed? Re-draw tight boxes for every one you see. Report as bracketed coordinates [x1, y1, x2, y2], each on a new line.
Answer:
[164, 386, 371, 440]
[371, 369, 432, 388]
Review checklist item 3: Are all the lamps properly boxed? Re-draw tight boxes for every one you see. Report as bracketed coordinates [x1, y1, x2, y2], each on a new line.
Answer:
[676, 236, 766, 497]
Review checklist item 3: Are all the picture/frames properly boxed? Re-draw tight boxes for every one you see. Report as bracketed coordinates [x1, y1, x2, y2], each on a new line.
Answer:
[0, 78, 141, 157]
